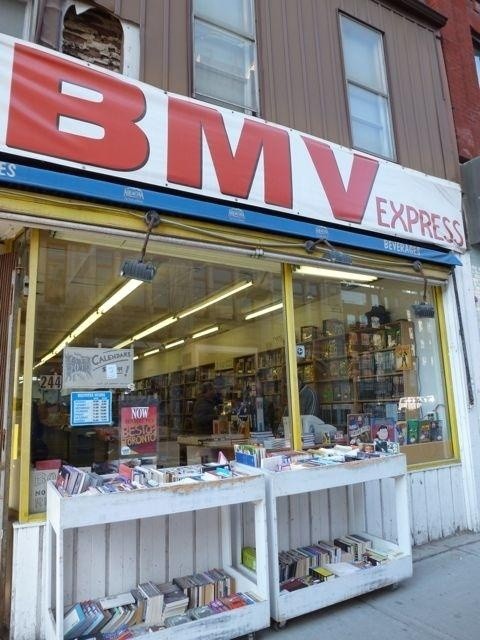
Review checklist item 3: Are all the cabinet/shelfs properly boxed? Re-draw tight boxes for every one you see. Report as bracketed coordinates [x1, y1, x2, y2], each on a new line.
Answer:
[132, 321, 420, 442]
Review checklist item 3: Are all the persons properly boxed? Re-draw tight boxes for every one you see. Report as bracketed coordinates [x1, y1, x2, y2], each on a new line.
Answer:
[30, 402, 52, 467]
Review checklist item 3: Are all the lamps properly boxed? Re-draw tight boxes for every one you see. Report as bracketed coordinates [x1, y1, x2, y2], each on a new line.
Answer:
[120, 209, 163, 283]
[410, 259, 438, 319]
[304, 234, 354, 266]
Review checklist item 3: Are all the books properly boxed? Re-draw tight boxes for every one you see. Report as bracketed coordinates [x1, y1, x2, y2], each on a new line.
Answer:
[56, 567, 263, 638]
[126, 311, 445, 441]
[243, 534, 392, 594]
[54, 438, 404, 500]
